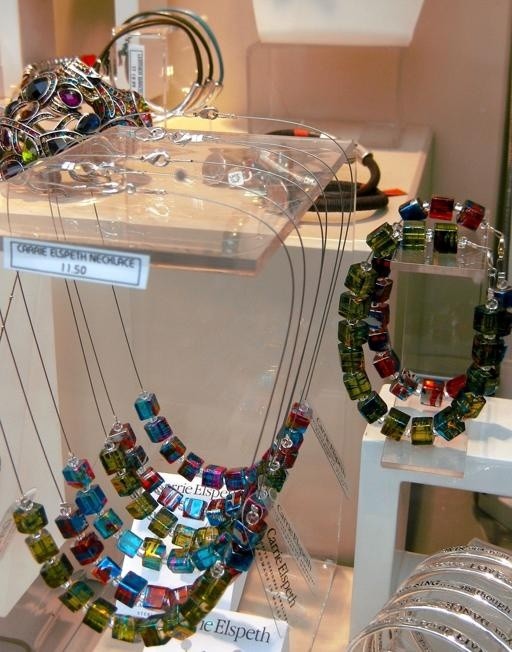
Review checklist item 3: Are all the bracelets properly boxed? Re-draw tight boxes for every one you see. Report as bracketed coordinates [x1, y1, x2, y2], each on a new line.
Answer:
[348, 545, 512, 652]
[260, 128, 381, 199]
[274, 180, 388, 212]
